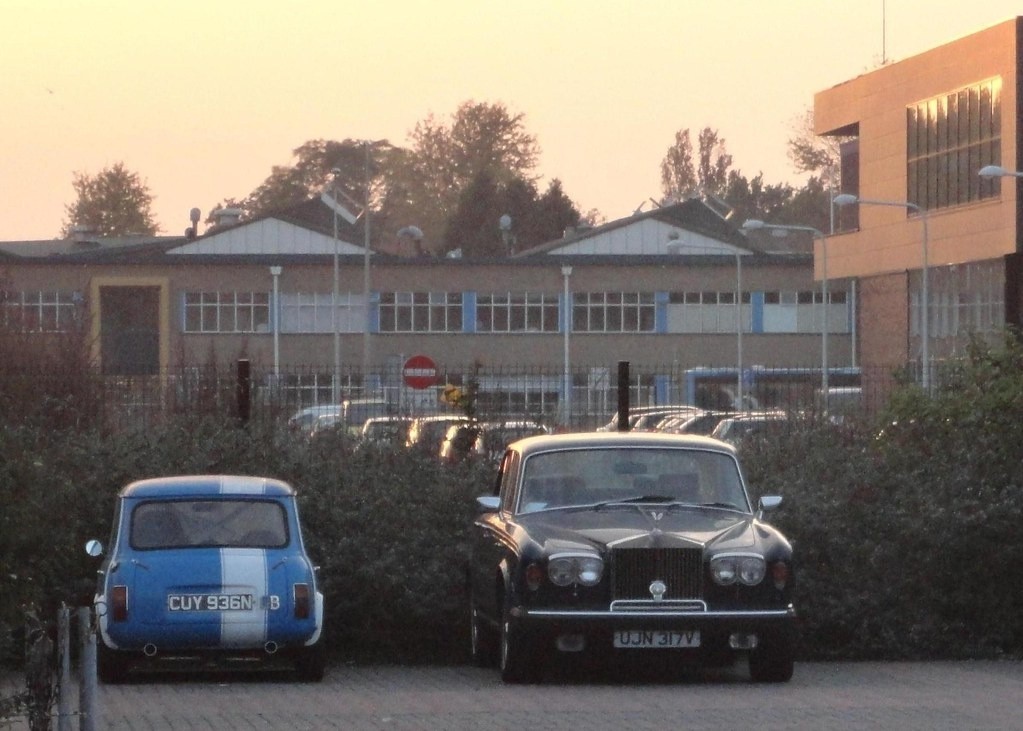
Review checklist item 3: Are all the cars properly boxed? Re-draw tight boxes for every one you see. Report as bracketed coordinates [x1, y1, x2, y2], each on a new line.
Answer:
[86, 472, 327, 688]
[285, 400, 901, 496]
[461, 429, 801, 687]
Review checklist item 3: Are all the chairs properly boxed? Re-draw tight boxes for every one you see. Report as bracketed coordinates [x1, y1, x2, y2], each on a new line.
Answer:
[533, 469, 705, 505]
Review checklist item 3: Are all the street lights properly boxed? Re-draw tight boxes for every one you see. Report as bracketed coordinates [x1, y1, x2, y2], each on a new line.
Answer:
[741, 216, 828, 403]
[666, 236, 743, 411]
[833, 194, 930, 399]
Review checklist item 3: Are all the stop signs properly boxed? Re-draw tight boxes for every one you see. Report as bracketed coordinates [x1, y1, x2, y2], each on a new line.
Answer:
[402, 357, 439, 390]
